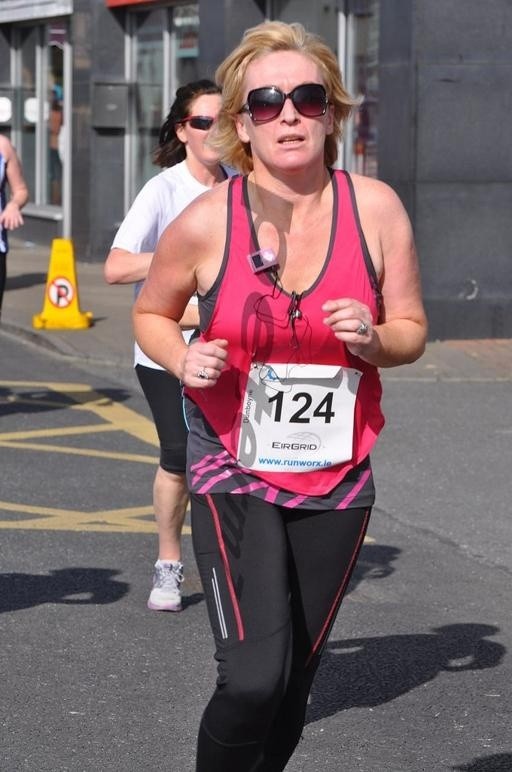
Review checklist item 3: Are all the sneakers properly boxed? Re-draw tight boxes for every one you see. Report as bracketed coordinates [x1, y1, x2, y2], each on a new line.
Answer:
[147, 561, 184, 611]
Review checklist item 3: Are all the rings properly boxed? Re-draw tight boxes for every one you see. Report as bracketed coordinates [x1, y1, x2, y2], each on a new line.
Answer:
[357, 322, 368, 336]
[196, 368, 208, 379]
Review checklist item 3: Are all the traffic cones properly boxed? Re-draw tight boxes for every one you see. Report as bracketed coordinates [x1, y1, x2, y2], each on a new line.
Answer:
[32, 238, 93, 330]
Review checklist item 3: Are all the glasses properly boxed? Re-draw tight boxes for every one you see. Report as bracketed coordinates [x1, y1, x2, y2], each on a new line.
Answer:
[181, 116, 217, 130]
[238, 84, 329, 121]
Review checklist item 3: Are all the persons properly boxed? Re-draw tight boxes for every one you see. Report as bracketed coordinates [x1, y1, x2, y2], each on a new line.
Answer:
[131, 20, 428, 771]
[0, 129, 30, 306]
[104, 81, 249, 613]
